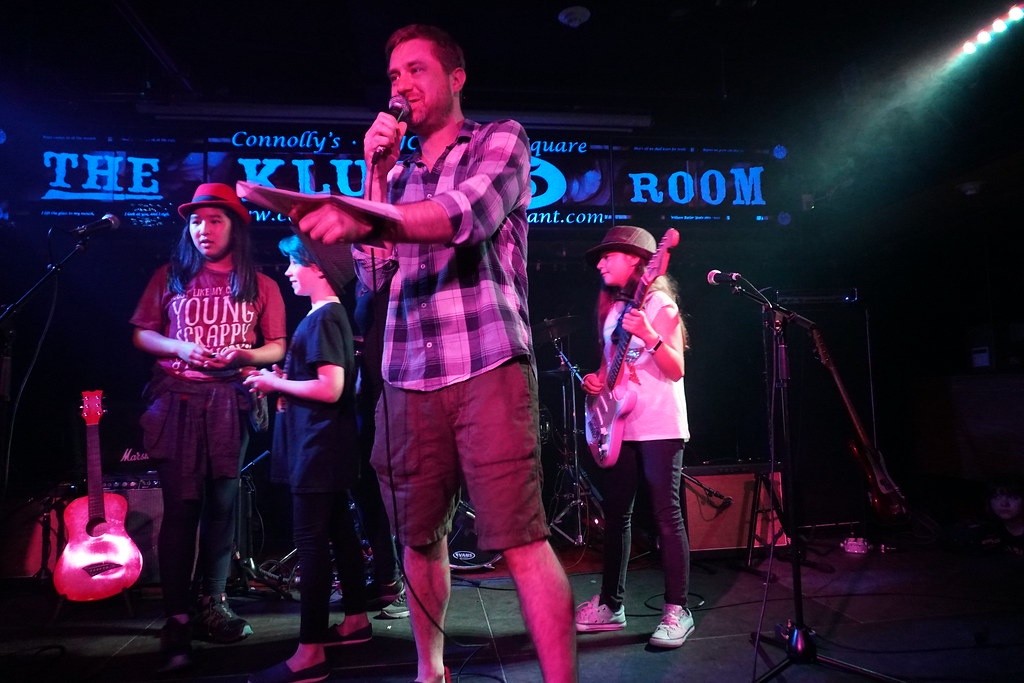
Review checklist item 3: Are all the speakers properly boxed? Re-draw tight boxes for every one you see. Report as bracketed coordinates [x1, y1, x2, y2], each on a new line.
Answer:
[0, 474, 162, 591]
[679, 464, 794, 558]
[747, 299, 880, 531]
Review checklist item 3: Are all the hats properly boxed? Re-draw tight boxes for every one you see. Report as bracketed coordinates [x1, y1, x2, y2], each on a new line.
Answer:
[178, 183, 251, 225]
[288, 226, 357, 297]
[586, 226, 657, 261]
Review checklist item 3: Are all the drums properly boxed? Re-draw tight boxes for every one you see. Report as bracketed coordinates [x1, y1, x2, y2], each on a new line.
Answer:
[447, 474, 503, 569]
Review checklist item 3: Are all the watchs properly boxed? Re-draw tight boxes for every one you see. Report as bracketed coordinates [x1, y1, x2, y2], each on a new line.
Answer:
[643, 334, 664, 356]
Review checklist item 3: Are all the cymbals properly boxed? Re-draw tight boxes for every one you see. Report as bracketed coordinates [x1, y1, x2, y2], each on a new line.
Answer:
[531, 315, 587, 348]
[537, 366, 593, 380]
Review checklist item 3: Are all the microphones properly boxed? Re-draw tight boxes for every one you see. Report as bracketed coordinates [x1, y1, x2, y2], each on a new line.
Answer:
[67, 214, 119, 237]
[722, 496, 733, 506]
[371, 95, 411, 165]
[708, 269, 742, 285]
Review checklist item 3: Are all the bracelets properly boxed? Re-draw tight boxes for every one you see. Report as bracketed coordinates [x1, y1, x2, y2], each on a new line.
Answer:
[582, 373, 597, 387]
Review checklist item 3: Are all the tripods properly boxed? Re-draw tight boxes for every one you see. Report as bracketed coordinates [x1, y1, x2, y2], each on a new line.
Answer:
[541, 327, 603, 550]
[728, 281, 900, 683]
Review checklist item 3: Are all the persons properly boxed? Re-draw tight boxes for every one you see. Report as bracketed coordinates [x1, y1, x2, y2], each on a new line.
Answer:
[285, 18, 581, 683]
[581, 226, 696, 651]
[242, 222, 372, 683]
[127, 184, 287, 677]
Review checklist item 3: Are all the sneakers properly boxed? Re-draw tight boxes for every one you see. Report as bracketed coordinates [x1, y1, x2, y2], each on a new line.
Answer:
[340, 581, 406, 606]
[156, 618, 195, 672]
[650, 604, 696, 647]
[381, 592, 410, 618]
[575, 595, 627, 632]
[192, 592, 254, 644]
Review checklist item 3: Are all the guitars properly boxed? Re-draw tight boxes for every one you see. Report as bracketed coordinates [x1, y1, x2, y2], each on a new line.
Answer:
[584, 228, 680, 469]
[52, 390, 143, 604]
[807, 327, 909, 522]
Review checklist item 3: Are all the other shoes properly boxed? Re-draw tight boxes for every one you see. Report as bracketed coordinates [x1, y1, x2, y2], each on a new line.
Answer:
[297, 623, 373, 646]
[247, 661, 330, 683]
